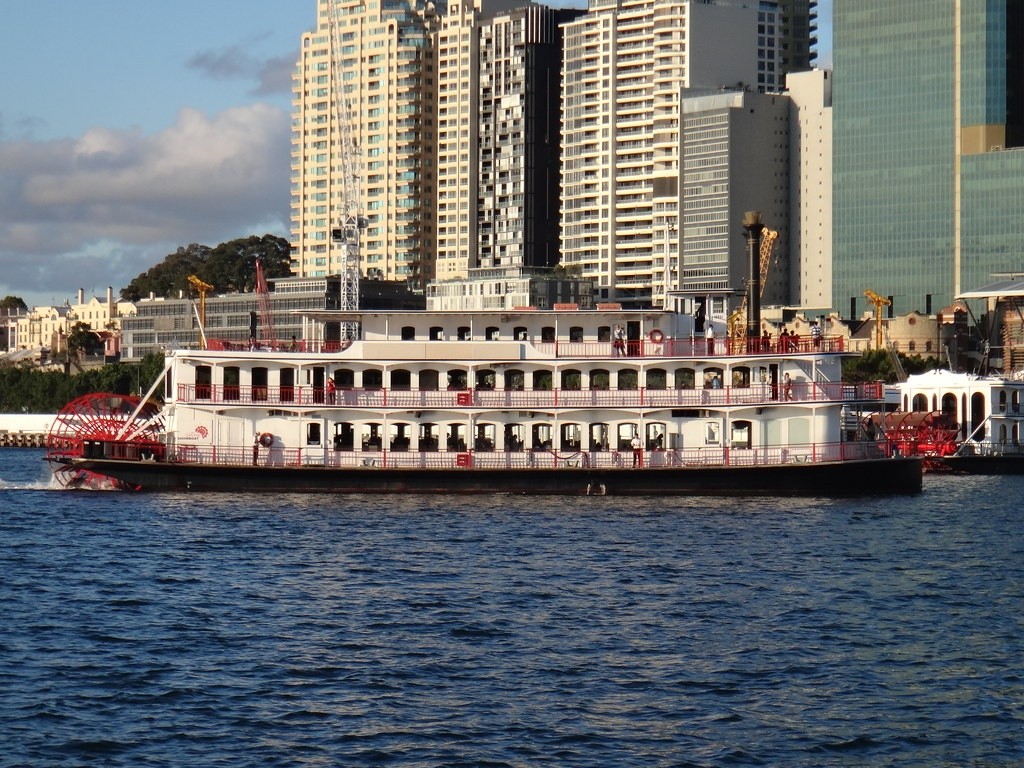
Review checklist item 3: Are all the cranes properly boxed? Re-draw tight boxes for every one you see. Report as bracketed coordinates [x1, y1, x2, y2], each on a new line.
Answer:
[865, 291, 891, 349]
[187, 275, 215, 351]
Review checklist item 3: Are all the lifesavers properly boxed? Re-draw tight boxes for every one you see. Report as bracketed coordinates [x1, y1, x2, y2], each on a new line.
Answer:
[648, 328, 665, 344]
[260, 433, 274, 447]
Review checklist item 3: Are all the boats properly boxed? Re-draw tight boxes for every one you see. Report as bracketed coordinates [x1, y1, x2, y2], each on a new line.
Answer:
[878, 367, 1024, 475]
[40, 307, 923, 496]
